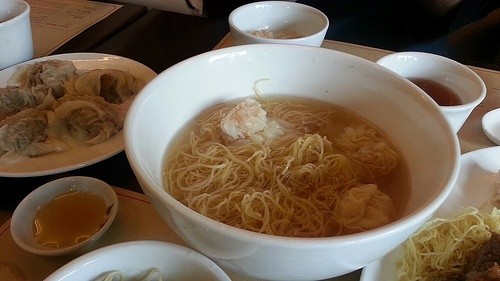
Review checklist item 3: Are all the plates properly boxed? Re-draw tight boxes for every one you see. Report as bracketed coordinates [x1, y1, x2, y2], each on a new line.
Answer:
[0, 53, 159, 178]
[43, 240, 234, 281]
[481, 107, 500, 144]
[359, 145, 500, 281]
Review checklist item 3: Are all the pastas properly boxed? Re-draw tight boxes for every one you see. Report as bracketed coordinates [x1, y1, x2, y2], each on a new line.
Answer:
[392, 205, 500, 281]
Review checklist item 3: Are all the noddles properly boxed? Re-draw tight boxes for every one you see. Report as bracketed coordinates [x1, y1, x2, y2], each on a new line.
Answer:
[160, 77, 411, 237]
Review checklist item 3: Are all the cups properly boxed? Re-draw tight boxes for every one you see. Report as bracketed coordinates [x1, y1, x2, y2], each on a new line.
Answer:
[0, 0, 34, 70]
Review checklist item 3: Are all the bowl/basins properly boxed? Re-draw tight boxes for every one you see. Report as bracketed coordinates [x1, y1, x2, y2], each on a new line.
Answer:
[10, 176, 120, 256]
[123, 43, 461, 281]
[229, 1, 329, 47]
[375, 51, 487, 135]
